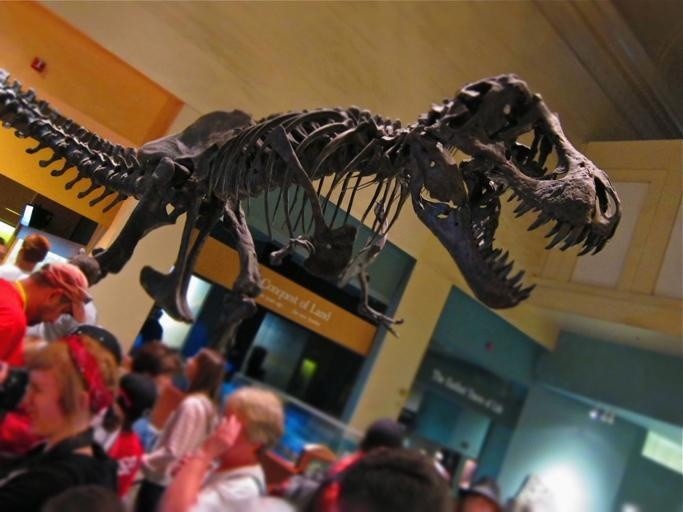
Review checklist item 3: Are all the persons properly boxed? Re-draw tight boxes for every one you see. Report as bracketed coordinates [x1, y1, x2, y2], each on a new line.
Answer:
[1, 234, 510, 512]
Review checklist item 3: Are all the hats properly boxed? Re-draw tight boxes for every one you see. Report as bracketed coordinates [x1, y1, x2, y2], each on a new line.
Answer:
[71, 325, 122, 365]
[366, 418, 405, 450]
[40, 263, 93, 323]
[459, 476, 503, 509]
[117, 373, 157, 416]
